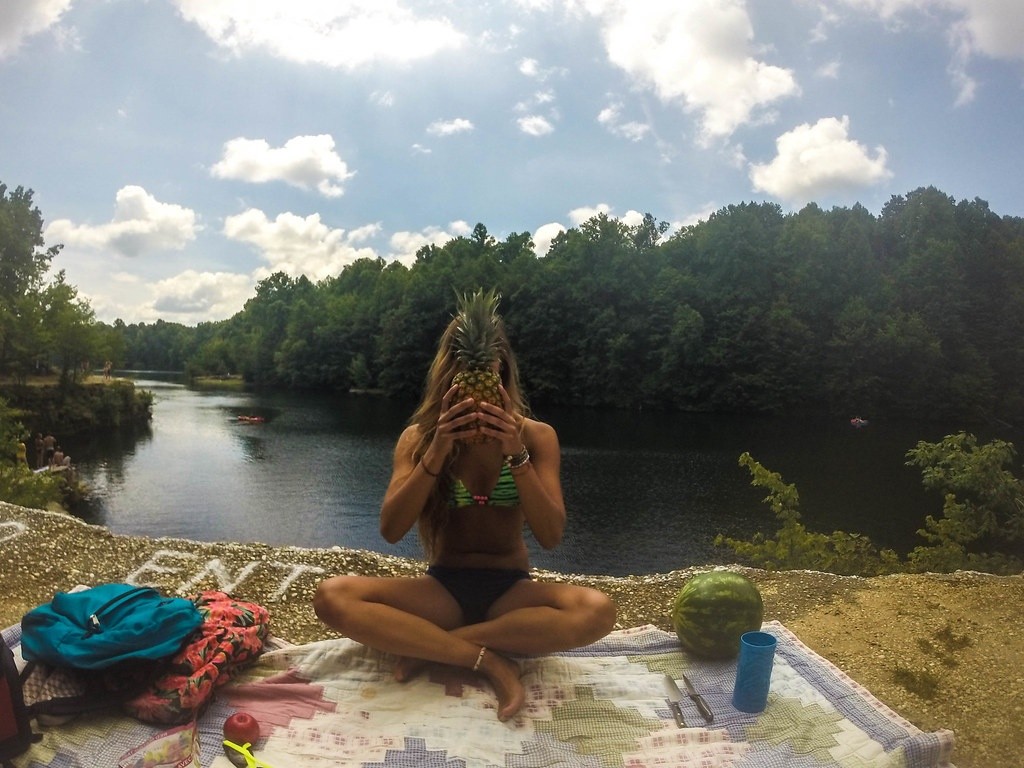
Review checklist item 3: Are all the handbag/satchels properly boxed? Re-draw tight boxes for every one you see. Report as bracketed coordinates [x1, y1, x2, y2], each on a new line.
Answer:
[21, 583, 202, 671]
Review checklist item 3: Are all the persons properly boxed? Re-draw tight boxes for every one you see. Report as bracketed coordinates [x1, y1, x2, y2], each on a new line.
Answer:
[313, 313, 616, 723]
[103, 360, 113, 379]
[17, 432, 67, 471]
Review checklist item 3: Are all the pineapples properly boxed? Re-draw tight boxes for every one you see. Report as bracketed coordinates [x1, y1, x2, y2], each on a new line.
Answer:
[451, 286, 504, 445]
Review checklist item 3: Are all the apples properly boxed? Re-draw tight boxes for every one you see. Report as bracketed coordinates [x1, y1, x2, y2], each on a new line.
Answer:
[223, 713, 260, 747]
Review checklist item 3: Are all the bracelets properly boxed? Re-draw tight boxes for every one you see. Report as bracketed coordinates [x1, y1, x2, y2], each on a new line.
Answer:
[420, 455, 440, 478]
[503, 445, 531, 469]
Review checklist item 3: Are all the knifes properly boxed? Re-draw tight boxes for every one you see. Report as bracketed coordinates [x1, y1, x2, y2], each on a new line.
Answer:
[682, 674, 714, 723]
[662, 675, 685, 728]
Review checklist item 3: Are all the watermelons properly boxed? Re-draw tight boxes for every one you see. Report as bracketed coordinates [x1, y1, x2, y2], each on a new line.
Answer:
[673, 571, 764, 658]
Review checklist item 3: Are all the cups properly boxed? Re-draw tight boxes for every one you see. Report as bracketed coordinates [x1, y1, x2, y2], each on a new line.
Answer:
[732, 631, 778, 713]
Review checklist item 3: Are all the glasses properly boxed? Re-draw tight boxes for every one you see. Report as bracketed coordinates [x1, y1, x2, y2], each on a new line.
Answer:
[222, 740, 272, 768]
